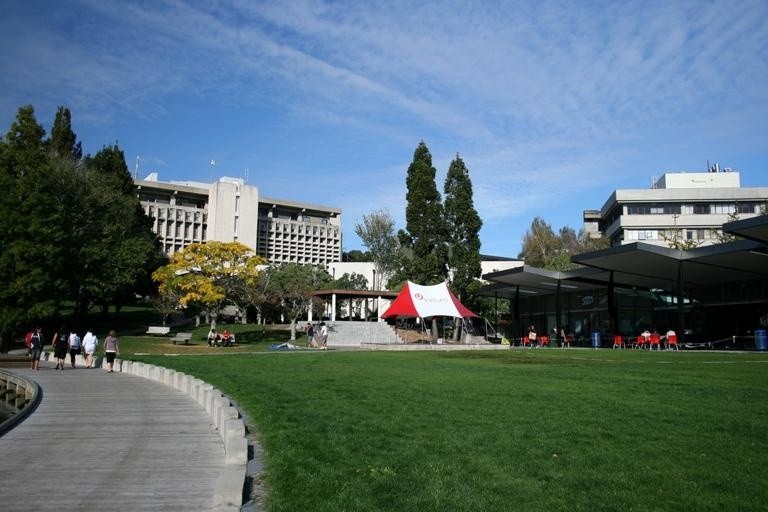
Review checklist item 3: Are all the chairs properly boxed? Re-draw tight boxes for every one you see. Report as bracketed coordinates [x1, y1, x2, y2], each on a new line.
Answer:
[520, 334, 678, 352]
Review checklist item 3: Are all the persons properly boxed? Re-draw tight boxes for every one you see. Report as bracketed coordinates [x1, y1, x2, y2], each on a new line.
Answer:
[319, 322, 327, 348]
[306, 323, 313, 348]
[103, 329, 119, 372]
[25, 325, 98, 370]
[641, 327, 676, 350]
[528, 324, 565, 349]
[208, 328, 218, 347]
[222, 328, 231, 346]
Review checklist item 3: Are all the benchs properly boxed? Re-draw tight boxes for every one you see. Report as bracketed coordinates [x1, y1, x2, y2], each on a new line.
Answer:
[169, 332, 193, 345]
[146, 326, 171, 337]
[211, 333, 236, 347]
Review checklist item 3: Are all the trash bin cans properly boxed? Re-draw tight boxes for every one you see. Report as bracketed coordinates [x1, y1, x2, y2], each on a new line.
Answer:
[592, 331, 601, 347]
[755, 330, 768, 350]
